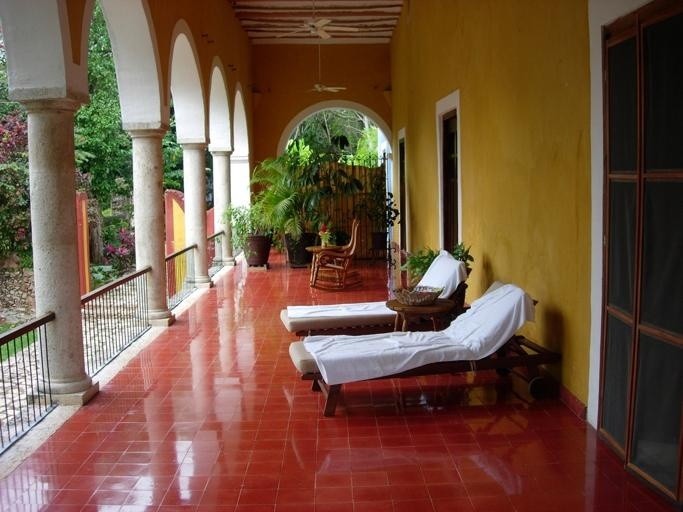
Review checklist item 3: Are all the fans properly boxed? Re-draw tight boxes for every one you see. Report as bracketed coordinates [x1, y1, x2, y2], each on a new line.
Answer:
[277, 0, 368, 41]
[312, 84, 347, 93]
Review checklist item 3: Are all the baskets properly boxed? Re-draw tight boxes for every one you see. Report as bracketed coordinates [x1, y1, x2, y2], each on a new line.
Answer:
[393, 286, 443, 305]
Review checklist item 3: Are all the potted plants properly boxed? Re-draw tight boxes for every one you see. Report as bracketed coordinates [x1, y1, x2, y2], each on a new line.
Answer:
[353, 171, 402, 258]
[226, 207, 272, 266]
[250, 150, 320, 268]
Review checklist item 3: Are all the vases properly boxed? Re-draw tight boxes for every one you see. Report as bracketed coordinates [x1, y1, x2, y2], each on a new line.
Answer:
[320, 237, 328, 246]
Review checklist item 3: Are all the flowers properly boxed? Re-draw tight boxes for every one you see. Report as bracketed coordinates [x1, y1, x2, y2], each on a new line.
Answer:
[318, 221, 337, 241]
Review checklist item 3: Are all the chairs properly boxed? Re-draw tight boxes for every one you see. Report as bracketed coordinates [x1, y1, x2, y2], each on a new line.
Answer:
[288, 280, 561, 417]
[280, 250, 471, 340]
[312, 218, 361, 289]
